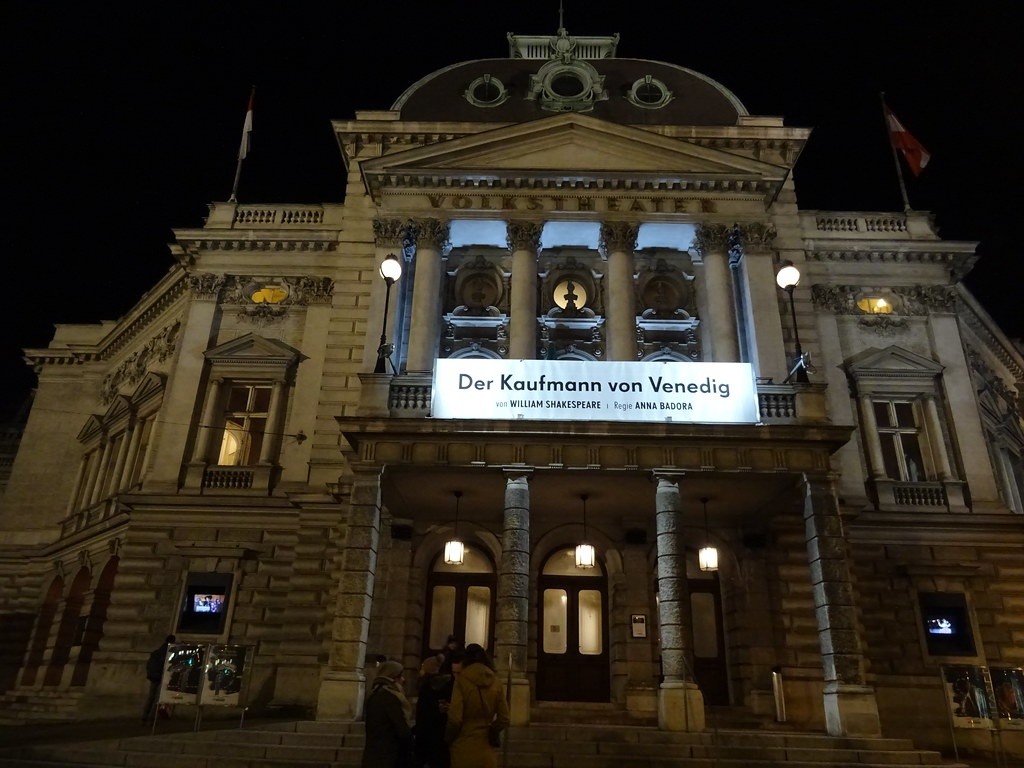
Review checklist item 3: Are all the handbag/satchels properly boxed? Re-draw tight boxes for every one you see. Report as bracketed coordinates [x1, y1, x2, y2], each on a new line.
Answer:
[487, 725, 500, 747]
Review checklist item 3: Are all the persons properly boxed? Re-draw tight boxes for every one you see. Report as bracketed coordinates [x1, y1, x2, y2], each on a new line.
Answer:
[413, 654, 451, 768]
[441, 635, 462, 661]
[936, 618, 952, 634]
[361, 661, 418, 768]
[200, 595, 219, 613]
[141, 633, 175, 723]
[445, 645, 510, 768]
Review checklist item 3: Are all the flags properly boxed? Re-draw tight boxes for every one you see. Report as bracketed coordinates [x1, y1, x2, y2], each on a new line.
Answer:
[883, 100, 931, 178]
[236, 91, 254, 161]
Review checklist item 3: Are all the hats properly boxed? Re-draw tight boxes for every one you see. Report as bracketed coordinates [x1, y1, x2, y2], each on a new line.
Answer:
[376, 661, 403, 679]
[447, 634, 456, 645]
[422, 656, 436, 672]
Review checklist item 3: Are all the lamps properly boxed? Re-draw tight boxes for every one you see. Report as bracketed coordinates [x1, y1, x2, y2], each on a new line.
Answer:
[443, 488, 465, 565]
[698, 499, 720, 571]
[574, 492, 596, 569]
